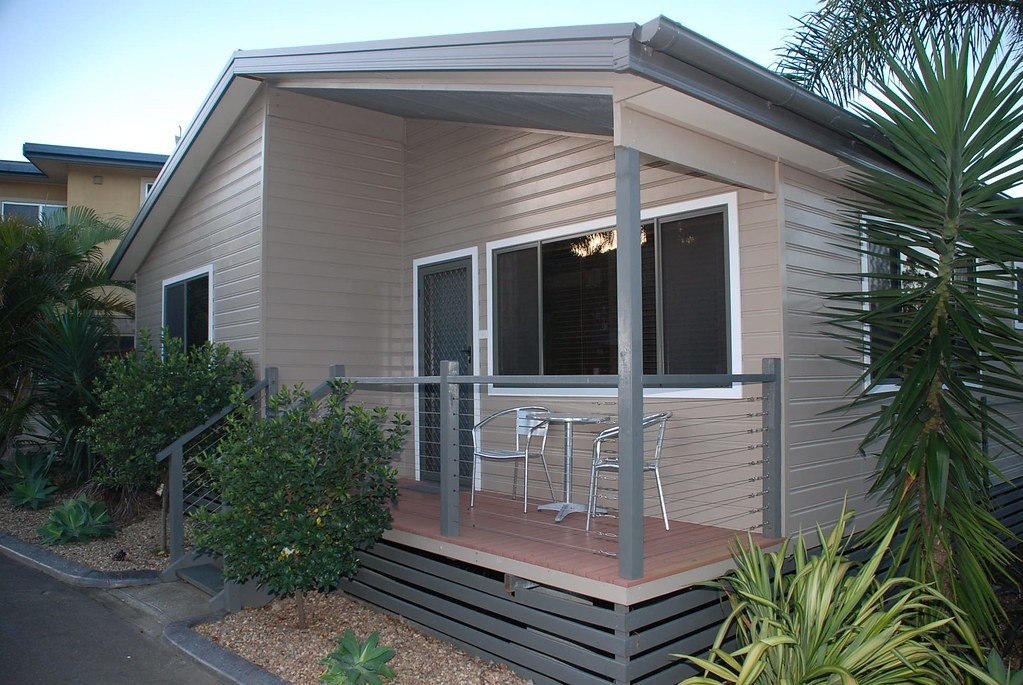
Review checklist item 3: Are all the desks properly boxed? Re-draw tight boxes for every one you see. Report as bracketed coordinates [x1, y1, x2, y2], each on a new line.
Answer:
[525, 413, 610, 524]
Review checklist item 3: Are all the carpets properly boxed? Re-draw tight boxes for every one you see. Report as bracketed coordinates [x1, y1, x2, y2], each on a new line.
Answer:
[399, 482, 472, 495]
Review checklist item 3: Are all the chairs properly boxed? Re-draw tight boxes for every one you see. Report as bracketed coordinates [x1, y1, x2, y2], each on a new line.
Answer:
[470, 406, 557, 514]
[585, 410, 673, 531]
